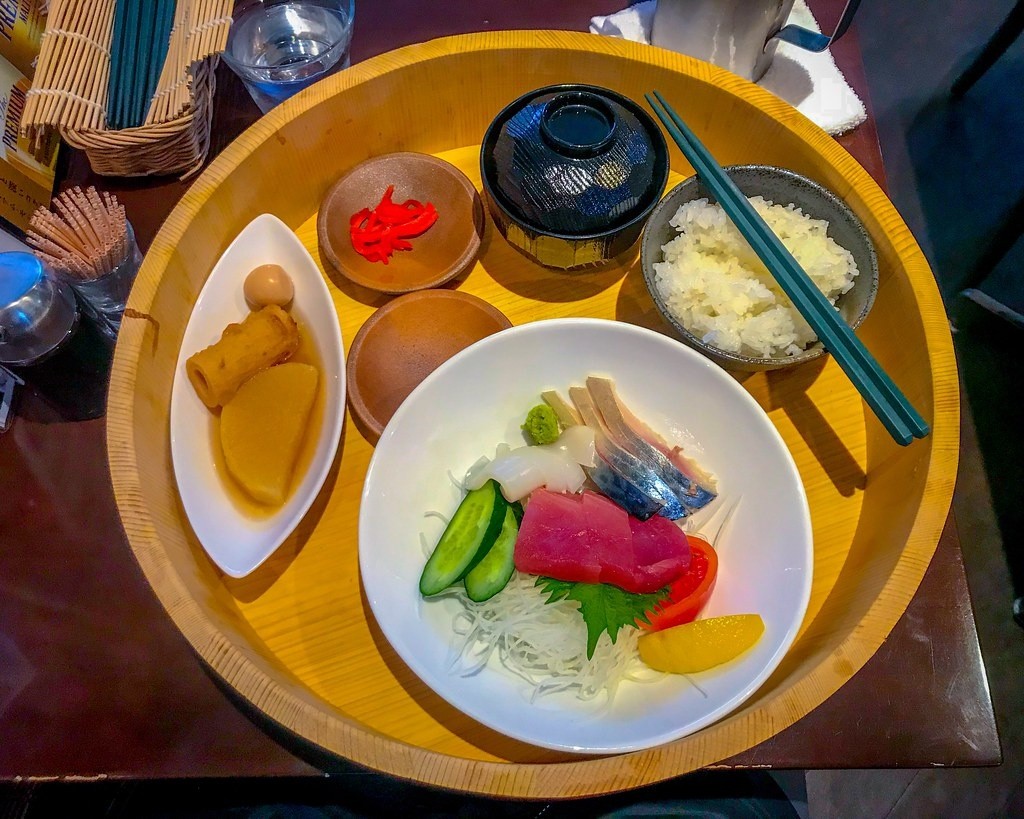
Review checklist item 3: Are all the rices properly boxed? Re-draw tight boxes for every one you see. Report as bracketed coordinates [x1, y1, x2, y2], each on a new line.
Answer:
[652, 197, 859, 357]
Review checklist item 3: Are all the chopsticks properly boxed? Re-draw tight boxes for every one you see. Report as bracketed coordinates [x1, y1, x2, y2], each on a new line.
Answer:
[644, 88, 929, 450]
[105, 0, 180, 129]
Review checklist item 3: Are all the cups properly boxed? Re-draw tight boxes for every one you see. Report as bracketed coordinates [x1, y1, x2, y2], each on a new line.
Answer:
[220, 0, 356, 115]
[69, 219, 144, 331]
[649, 0, 862, 84]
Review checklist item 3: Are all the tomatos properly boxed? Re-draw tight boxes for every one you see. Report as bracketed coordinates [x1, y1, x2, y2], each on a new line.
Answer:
[632, 535, 720, 631]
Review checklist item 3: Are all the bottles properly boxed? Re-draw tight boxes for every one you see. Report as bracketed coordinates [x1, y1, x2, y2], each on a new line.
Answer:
[0, 251, 119, 422]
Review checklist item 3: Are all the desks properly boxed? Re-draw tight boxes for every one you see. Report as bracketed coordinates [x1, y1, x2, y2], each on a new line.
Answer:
[0, 0, 1004, 790]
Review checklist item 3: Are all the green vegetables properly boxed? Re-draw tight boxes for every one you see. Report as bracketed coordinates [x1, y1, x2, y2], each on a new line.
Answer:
[530, 574, 673, 661]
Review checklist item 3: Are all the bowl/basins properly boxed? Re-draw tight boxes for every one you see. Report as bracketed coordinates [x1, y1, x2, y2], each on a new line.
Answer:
[639, 164, 878, 373]
[480, 80, 670, 271]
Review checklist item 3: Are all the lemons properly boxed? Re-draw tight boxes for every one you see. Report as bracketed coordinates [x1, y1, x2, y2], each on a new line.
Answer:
[637, 614, 766, 676]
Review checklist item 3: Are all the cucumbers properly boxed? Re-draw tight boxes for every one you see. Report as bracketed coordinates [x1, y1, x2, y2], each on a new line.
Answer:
[419, 481, 525, 602]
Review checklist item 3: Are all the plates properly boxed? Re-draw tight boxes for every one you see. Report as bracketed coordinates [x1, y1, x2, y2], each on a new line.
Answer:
[355, 316, 814, 756]
[170, 212, 347, 580]
[317, 151, 485, 296]
[345, 287, 513, 437]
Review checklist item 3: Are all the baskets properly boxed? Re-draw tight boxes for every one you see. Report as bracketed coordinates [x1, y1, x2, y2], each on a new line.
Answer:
[57, 54, 219, 183]
